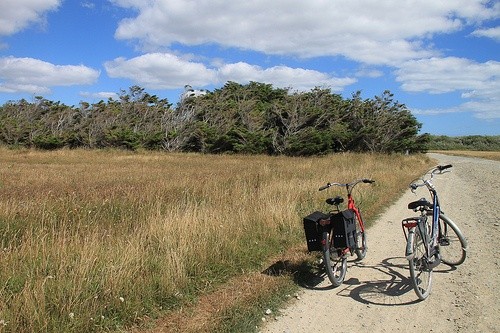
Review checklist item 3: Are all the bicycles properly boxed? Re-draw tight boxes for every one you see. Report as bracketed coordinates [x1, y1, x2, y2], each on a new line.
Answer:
[318, 178, 376, 284]
[402, 163, 468, 301]
